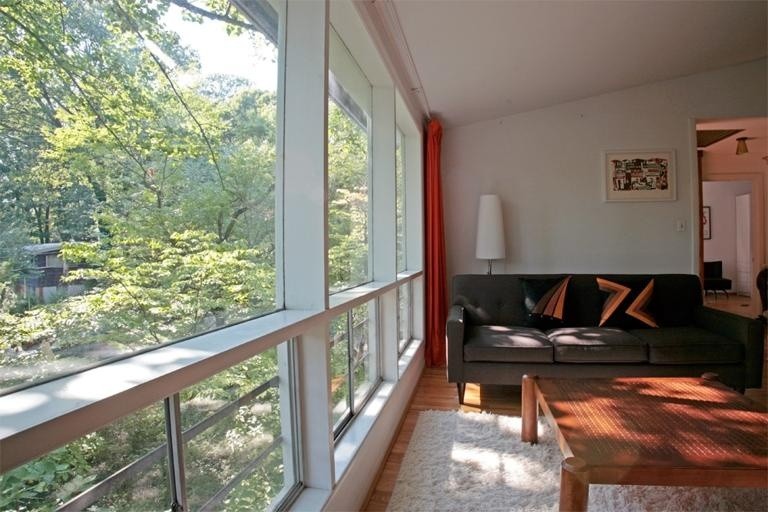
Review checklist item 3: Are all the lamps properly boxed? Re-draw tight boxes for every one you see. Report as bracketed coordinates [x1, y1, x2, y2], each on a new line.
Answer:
[736, 137, 748, 154]
[476, 194, 506, 274]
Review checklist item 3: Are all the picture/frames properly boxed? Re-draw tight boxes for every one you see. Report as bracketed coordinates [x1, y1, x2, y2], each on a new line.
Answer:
[703, 206, 711, 240]
[602, 149, 677, 201]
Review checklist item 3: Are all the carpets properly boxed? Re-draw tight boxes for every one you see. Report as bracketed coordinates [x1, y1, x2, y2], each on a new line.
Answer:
[387, 409, 768, 512]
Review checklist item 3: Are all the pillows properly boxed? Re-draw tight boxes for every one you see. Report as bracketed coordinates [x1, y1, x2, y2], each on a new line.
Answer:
[593, 274, 660, 330]
[519, 276, 573, 328]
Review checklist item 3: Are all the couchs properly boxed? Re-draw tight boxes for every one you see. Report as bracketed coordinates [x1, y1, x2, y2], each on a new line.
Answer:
[446, 274, 764, 406]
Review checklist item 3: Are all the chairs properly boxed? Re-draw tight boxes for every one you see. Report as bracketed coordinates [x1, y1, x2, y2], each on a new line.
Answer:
[756, 267, 768, 316]
[704, 261, 731, 300]
